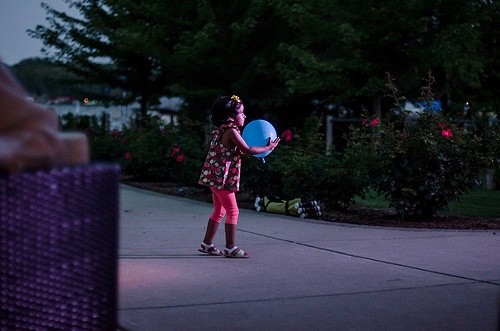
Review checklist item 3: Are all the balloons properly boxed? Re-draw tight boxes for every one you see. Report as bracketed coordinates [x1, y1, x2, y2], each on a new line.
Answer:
[241, 119, 277, 158]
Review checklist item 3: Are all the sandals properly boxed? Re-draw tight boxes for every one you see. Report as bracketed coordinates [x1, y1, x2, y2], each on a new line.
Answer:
[223, 245, 250, 258]
[197, 241, 222, 256]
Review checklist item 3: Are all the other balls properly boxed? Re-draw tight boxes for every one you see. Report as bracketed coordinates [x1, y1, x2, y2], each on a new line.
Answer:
[242, 120, 277, 157]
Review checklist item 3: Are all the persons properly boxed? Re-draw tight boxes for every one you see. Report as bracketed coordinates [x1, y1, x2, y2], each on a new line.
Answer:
[197, 94, 282, 258]
